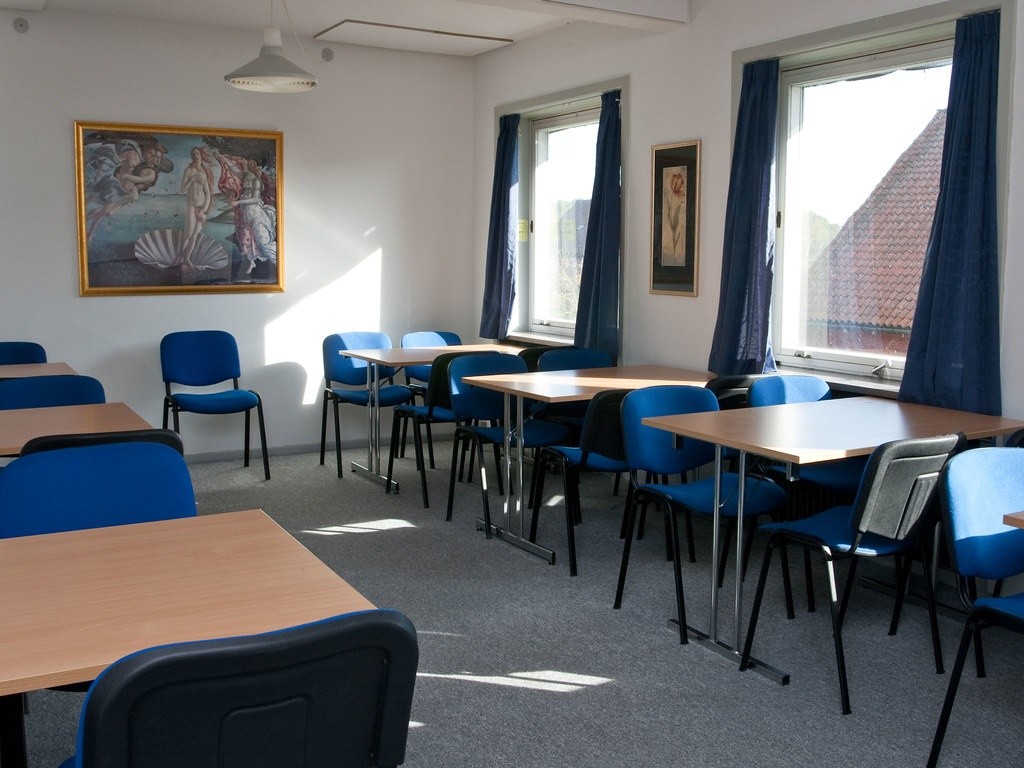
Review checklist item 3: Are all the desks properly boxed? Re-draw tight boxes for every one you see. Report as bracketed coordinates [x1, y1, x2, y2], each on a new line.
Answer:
[641, 396, 1024, 686]
[0, 509, 377, 768]
[0, 362, 80, 379]
[338, 343, 528, 496]
[0, 401, 156, 467]
[461, 363, 718, 565]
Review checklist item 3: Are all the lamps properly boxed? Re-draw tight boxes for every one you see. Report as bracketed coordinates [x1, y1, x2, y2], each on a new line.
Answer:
[225, 0, 321, 92]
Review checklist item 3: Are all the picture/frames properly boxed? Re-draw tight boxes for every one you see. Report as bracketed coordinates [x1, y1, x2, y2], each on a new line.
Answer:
[648, 139, 702, 298]
[73, 119, 285, 296]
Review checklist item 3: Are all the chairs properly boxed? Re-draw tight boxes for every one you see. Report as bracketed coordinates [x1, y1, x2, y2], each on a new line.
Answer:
[0, 428, 198, 714]
[58, 609, 419, 768]
[0, 341, 47, 365]
[161, 331, 271, 481]
[319, 331, 1024, 768]
[0, 376, 105, 410]
